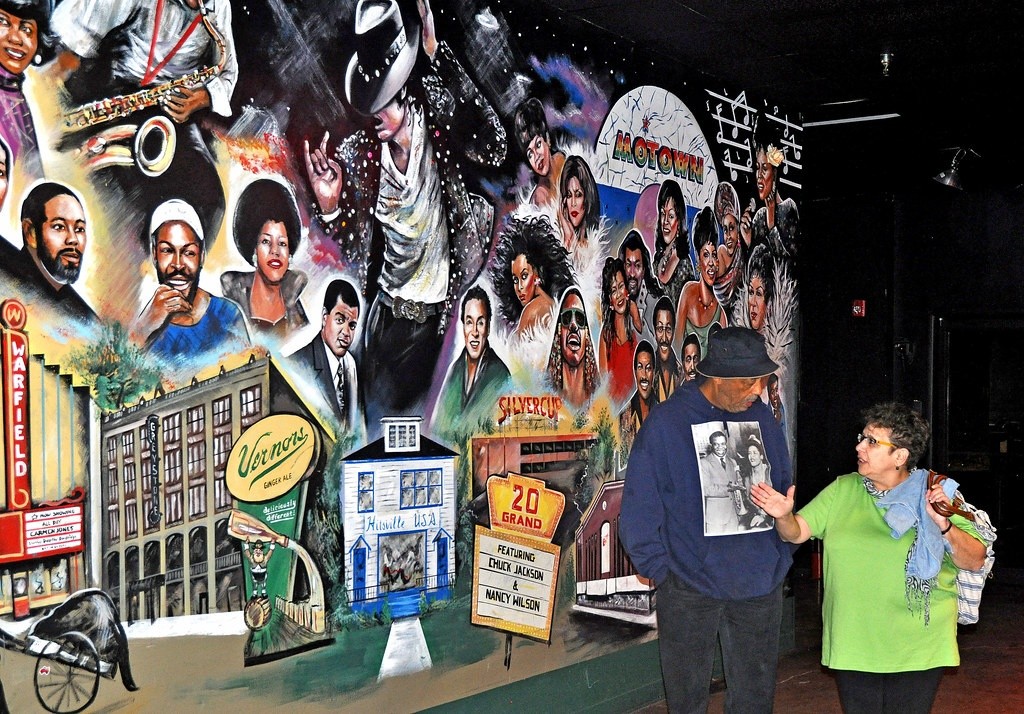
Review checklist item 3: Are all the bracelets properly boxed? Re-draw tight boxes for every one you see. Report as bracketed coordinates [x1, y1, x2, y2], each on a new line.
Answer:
[940, 517, 952, 534]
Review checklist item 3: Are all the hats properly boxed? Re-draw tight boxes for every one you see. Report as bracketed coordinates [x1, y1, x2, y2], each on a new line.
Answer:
[695, 327, 780, 379]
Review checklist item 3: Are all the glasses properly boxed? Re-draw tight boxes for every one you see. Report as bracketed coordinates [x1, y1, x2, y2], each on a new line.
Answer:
[857, 433, 902, 450]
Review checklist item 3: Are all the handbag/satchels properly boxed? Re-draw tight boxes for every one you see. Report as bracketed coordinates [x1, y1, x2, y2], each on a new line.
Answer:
[927, 469, 997, 625]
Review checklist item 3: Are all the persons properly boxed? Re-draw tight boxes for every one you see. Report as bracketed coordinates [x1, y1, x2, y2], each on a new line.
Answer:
[744, 404, 986, 714]
[623, 329, 795, 714]
